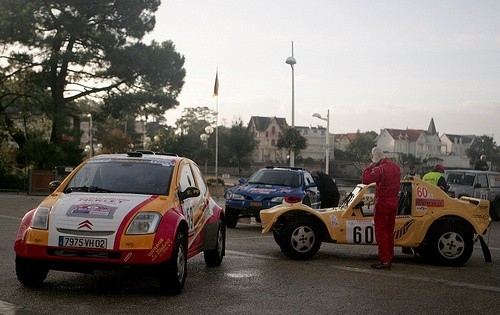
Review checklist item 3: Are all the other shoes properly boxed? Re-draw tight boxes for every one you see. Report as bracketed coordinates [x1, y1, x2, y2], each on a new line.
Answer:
[371, 260, 391, 269]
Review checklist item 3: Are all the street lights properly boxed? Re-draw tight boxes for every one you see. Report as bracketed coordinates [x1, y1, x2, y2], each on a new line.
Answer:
[201, 125, 214, 175]
[312, 110, 329, 173]
[285, 40, 296, 168]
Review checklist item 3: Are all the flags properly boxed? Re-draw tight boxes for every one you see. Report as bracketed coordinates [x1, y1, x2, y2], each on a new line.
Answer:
[213, 74, 219, 96]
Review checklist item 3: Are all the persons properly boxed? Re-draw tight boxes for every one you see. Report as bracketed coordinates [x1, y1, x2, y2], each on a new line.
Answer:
[311, 169, 340, 208]
[362, 147, 401, 269]
[475, 155, 489, 170]
[422, 164, 448, 192]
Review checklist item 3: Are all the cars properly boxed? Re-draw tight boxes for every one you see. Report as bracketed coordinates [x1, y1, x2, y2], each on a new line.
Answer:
[222, 165, 322, 228]
[13, 149, 226, 297]
[259, 175, 494, 264]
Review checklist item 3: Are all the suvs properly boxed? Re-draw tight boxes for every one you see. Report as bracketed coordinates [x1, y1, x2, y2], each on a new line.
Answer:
[443, 169, 500, 221]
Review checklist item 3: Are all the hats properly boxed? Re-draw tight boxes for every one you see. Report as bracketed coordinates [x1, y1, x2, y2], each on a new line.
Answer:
[436, 164, 445, 174]
[371, 147, 385, 164]
